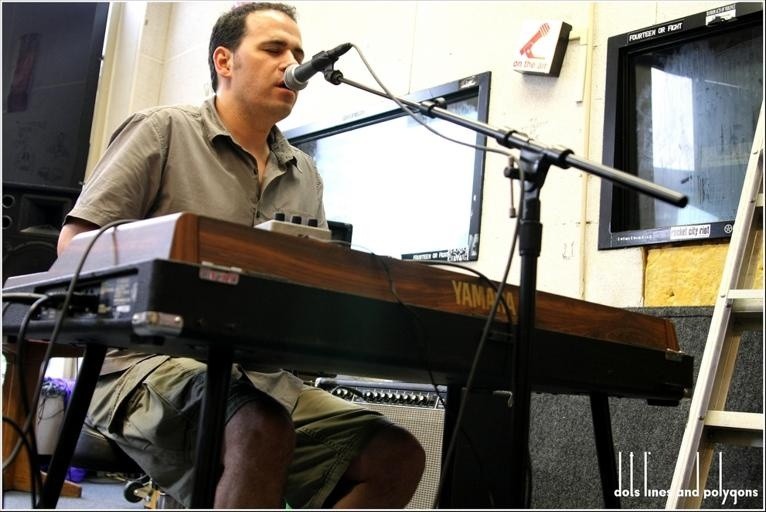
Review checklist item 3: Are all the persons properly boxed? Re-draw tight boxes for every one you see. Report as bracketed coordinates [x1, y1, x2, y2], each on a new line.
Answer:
[55, 2, 427, 510]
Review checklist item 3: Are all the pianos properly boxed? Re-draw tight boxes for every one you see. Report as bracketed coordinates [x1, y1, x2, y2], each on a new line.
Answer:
[2, 212, 693, 406]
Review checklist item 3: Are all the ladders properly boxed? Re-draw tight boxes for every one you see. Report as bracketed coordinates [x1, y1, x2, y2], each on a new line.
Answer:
[665, 98, 764, 510]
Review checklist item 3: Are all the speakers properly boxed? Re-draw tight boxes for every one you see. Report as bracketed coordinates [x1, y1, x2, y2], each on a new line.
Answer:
[2, 181, 82, 288]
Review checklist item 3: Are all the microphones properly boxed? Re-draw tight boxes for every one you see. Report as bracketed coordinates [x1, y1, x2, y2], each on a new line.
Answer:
[284, 41, 352, 92]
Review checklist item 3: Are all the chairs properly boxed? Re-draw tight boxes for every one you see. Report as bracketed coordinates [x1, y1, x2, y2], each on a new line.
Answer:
[34, 379, 186, 510]
